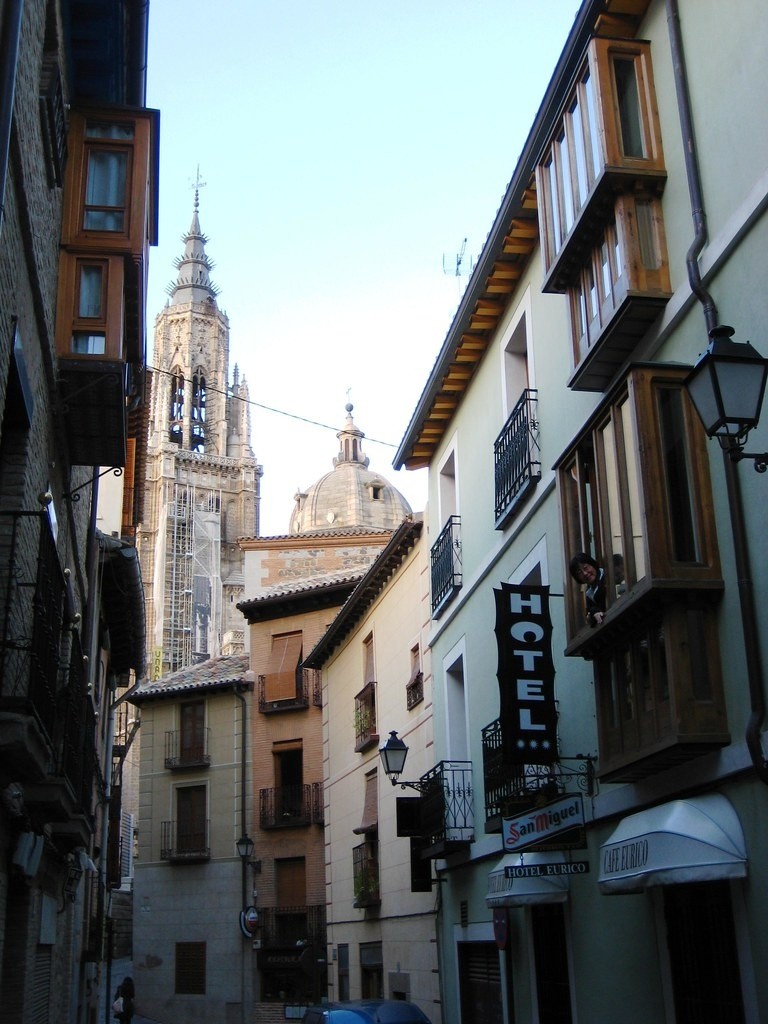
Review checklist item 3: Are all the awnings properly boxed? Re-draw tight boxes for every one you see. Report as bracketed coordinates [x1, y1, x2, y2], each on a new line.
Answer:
[485, 851, 568, 908]
[596, 793, 748, 899]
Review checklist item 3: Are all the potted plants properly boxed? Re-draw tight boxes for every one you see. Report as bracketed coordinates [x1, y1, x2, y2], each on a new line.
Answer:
[353, 858, 379, 902]
[357, 711, 376, 735]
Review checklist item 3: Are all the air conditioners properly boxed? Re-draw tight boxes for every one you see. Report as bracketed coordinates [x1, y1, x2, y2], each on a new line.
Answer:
[63, 867, 85, 896]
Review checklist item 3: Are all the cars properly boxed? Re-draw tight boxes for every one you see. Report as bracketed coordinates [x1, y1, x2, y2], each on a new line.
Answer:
[297, 1000, 433, 1024]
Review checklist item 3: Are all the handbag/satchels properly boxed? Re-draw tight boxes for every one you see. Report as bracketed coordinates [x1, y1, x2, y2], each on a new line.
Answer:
[112, 987, 124, 1014]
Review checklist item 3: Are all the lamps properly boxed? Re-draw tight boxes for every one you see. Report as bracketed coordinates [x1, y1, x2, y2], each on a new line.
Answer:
[236, 833, 261, 875]
[681, 324, 768, 474]
[378, 730, 443, 799]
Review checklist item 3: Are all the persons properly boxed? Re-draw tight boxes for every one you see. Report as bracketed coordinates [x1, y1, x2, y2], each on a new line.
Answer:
[113, 976, 135, 1024]
[568, 552, 608, 628]
